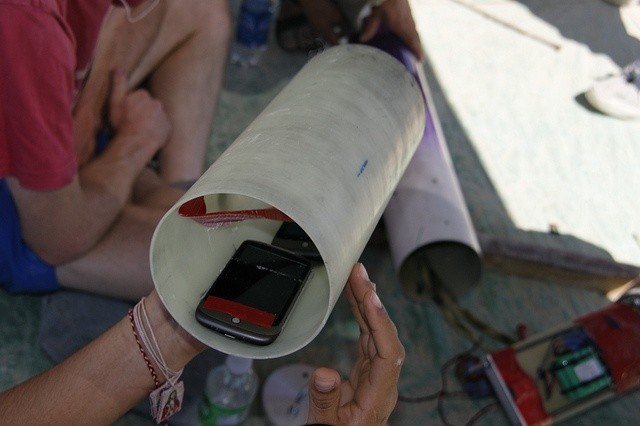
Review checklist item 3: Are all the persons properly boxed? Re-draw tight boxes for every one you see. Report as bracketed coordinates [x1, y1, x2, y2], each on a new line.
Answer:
[1, 259, 406, 426]
[1, 0, 232, 302]
[234, 0, 424, 62]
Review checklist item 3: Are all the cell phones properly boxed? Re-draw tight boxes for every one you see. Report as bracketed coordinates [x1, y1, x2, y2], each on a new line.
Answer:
[271, 222, 323, 264]
[195, 239, 313, 346]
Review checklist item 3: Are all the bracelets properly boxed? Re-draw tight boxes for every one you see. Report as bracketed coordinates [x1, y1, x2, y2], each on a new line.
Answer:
[125, 308, 161, 387]
[128, 295, 184, 422]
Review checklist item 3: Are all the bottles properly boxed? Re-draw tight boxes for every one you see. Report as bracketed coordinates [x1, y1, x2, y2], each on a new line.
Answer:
[196, 355, 260, 425]
